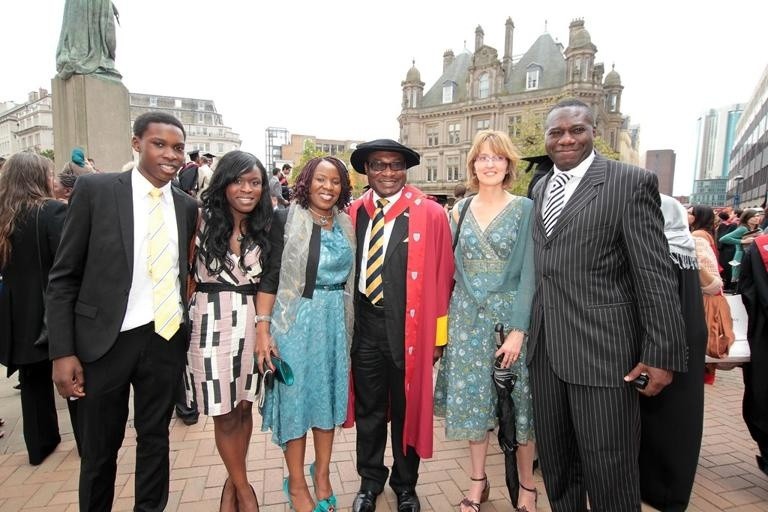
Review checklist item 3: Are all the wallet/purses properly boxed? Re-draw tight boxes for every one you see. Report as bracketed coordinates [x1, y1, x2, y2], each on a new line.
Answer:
[262, 360, 273, 389]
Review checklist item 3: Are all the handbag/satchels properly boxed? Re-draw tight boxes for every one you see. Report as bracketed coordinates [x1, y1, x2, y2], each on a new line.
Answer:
[270, 353, 295, 385]
[701, 292, 736, 360]
[741, 227, 763, 253]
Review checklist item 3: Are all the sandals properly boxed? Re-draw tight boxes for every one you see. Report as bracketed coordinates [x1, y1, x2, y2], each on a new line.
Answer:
[515, 481, 539, 512]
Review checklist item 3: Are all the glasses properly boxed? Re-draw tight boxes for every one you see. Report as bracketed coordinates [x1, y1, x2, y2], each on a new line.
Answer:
[475, 153, 505, 162]
[366, 156, 406, 171]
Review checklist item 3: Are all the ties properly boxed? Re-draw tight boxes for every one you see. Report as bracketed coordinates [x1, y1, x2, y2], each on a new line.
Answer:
[542, 171, 574, 238]
[146, 187, 183, 342]
[364, 199, 390, 308]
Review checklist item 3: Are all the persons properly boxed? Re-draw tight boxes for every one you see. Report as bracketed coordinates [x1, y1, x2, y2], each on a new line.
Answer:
[1, 100, 768, 511]
[56, 0, 122, 80]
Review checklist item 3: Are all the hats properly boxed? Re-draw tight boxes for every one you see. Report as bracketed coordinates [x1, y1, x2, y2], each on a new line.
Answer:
[188, 150, 217, 159]
[349, 138, 420, 175]
[519, 153, 554, 174]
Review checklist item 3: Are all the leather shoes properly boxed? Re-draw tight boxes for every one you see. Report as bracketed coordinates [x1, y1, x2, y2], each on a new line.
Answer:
[352, 485, 384, 512]
[219, 477, 259, 512]
[389, 478, 420, 512]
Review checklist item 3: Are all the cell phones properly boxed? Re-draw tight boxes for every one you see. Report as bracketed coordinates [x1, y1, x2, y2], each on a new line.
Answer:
[634, 373, 647, 389]
[262, 362, 274, 387]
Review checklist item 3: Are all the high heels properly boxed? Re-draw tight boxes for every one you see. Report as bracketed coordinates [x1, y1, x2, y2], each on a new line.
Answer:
[459, 473, 491, 512]
[281, 477, 322, 512]
[309, 462, 337, 512]
